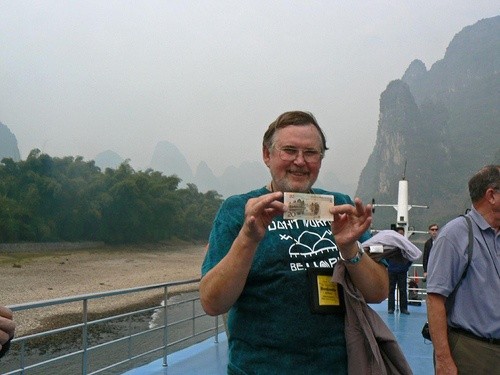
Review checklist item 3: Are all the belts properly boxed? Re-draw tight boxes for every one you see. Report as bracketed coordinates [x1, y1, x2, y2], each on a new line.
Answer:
[451, 326, 500, 346]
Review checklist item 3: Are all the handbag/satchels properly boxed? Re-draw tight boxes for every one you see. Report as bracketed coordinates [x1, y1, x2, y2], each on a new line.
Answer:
[422, 324, 432, 340]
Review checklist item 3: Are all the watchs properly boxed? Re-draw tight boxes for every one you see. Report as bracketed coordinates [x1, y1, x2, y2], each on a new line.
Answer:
[339, 239, 364, 265]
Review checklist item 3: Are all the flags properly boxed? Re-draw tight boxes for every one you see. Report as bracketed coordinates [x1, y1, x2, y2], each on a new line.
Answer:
[413, 268, 419, 284]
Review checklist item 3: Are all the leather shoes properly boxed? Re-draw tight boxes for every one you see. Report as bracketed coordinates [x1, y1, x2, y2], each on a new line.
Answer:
[388, 309, 394, 313]
[401, 308, 407, 313]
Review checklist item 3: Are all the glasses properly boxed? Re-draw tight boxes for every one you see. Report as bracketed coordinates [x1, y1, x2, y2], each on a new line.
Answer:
[431, 228, 438, 231]
[270, 142, 324, 164]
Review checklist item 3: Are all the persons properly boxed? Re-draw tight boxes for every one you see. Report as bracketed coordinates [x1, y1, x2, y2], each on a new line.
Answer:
[427, 165, 500, 375]
[199, 111, 389, 375]
[423, 224, 439, 280]
[385, 226, 413, 315]
